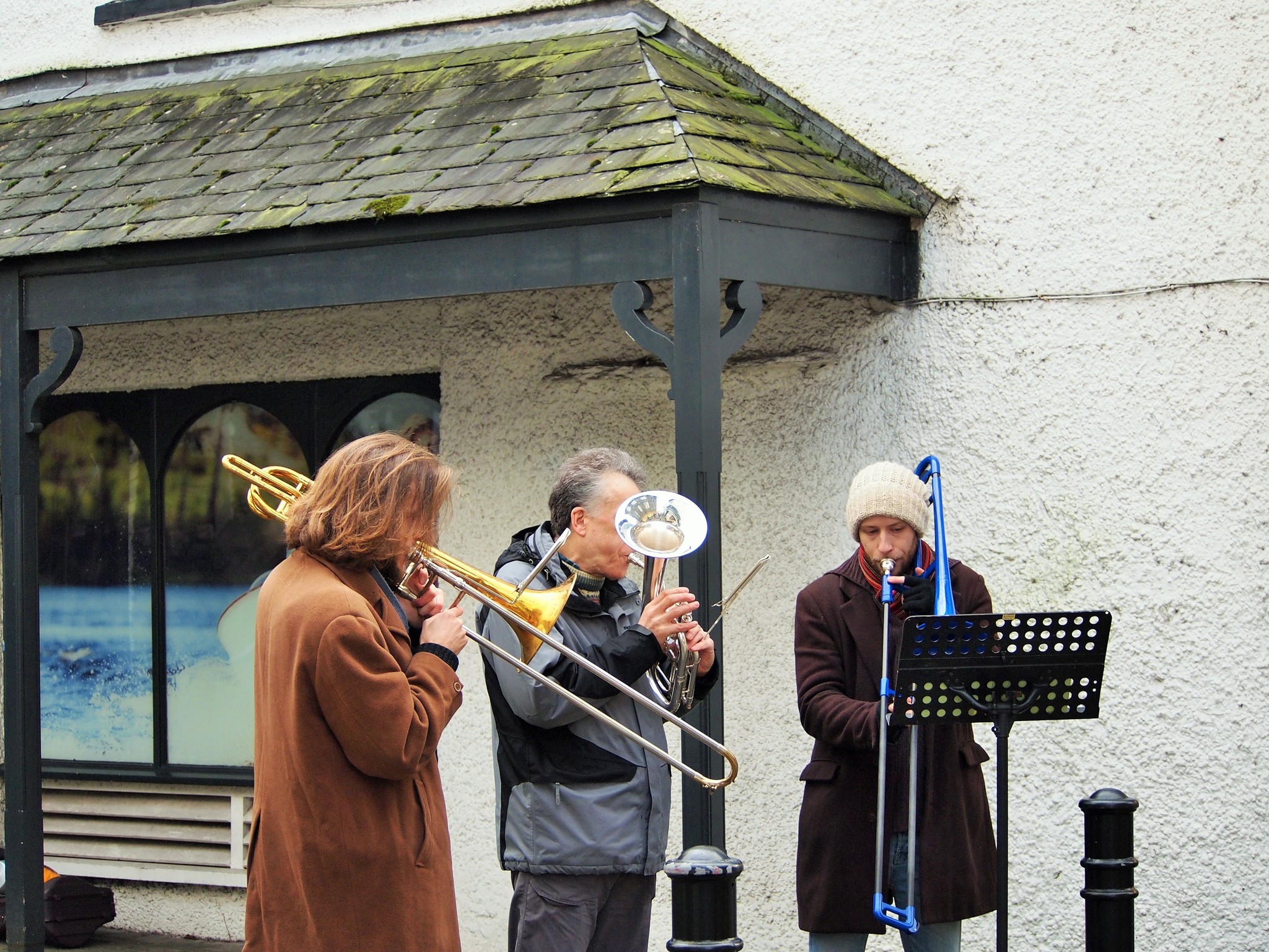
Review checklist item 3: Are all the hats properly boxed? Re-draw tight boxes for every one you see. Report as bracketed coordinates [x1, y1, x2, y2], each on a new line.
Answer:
[847, 460, 929, 539]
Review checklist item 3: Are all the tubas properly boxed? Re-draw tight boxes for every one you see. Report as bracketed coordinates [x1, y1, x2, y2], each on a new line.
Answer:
[613, 490, 709, 714]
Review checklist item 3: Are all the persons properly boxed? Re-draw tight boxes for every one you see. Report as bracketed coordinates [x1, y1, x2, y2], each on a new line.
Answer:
[794, 460, 1006, 952]
[482, 447, 719, 952]
[248, 433, 468, 952]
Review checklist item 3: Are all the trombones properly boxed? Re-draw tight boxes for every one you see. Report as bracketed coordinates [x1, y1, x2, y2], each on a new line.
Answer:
[222, 453, 740, 796]
[872, 454, 995, 935]
